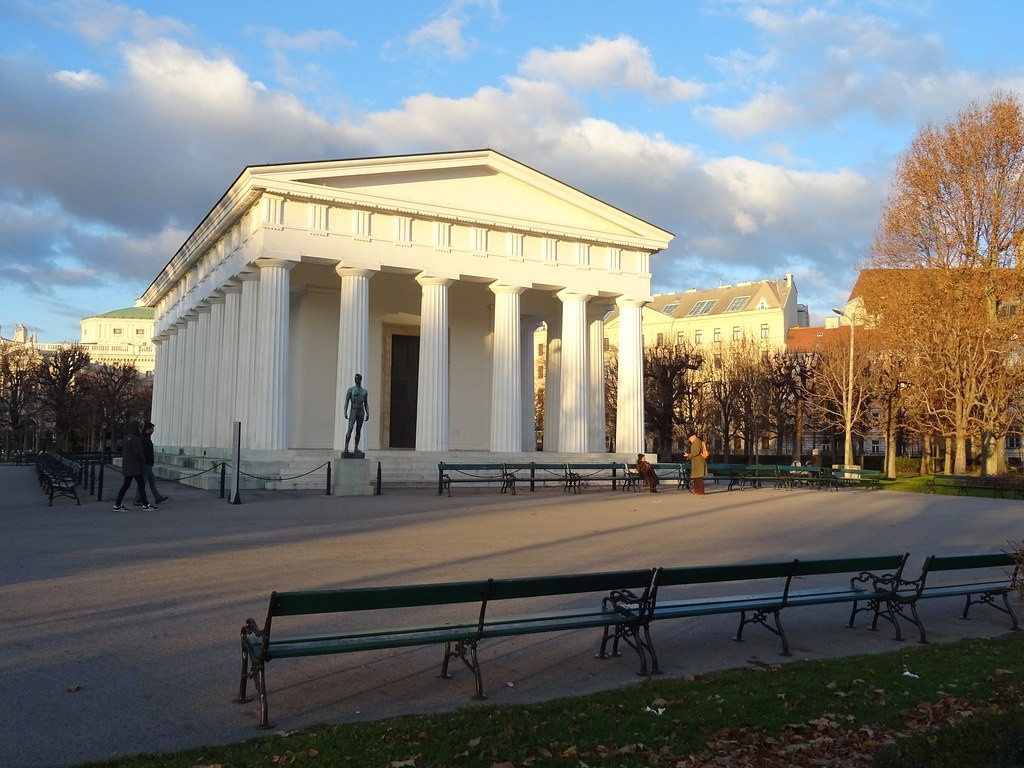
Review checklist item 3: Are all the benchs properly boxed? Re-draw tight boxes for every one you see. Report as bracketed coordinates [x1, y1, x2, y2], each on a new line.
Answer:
[438, 460, 883, 492]
[35, 450, 85, 507]
[232, 548, 1024, 731]
[925, 473, 1024, 501]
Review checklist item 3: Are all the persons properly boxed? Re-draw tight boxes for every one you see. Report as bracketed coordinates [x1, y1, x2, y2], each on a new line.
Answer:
[133, 422, 168, 506]
[636, 454, 659, 493]
[683, 433, 707, 495]
[112, 421, 159, 512]
[806, 448, 822, 467]
[344, 374, 369, 453]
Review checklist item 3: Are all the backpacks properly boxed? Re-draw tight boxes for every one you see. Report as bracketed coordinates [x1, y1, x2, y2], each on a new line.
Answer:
[700, 441, 709, 459]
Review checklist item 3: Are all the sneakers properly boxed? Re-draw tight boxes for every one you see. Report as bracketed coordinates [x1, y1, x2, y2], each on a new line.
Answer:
[113, 504, 128, 512]
[142, 503, 159, 511]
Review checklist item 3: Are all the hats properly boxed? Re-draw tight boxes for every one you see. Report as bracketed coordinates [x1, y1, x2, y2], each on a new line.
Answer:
[637, 453, 645, 461]
[685, 433, 694, 440]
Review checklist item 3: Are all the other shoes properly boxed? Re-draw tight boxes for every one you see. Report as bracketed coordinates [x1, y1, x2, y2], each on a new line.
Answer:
[690, 491, 704, 495]
[650, 489, 657, 493]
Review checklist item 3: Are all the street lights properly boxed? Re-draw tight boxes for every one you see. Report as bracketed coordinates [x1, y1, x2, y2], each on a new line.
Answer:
[832, 308, 853, 465]
[882, 379, 916, 481]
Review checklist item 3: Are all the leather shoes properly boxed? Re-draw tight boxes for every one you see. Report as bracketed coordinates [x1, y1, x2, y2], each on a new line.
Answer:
[133, 500, 142, 506]
[155, 496, 168, 503]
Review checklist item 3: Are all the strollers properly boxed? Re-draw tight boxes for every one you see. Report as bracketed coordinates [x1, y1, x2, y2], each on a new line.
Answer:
[789, 461, 812, 488]
